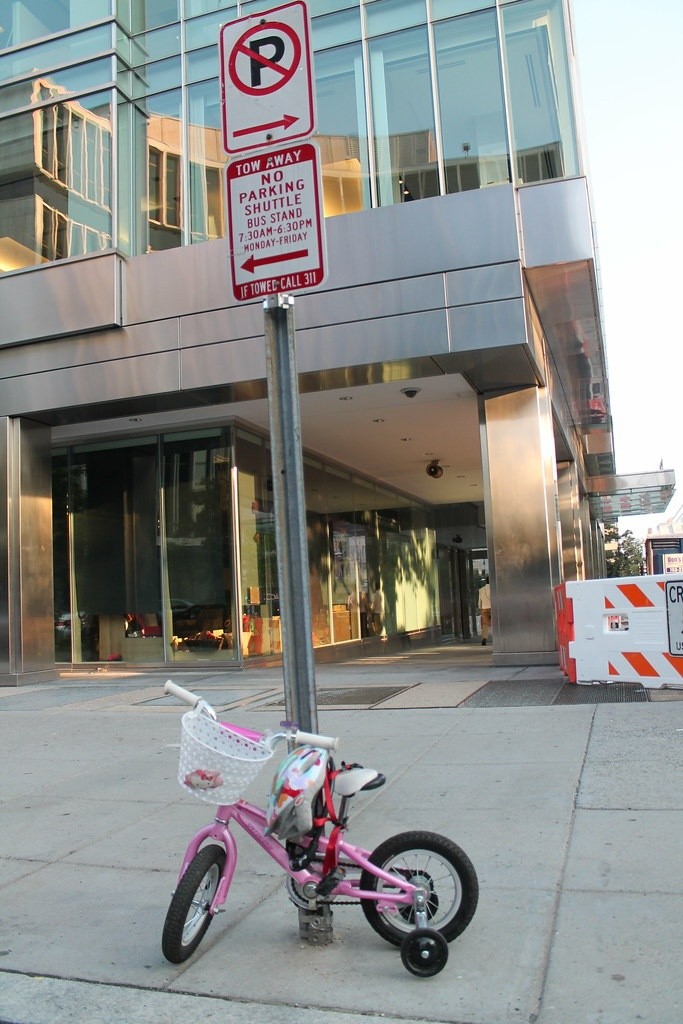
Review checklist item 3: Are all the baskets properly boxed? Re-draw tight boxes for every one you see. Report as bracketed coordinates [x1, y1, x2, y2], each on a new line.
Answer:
[176, 709, 274, 806]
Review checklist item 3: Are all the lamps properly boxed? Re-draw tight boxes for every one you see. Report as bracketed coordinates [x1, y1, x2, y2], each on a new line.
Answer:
[426, 460, 443, 478]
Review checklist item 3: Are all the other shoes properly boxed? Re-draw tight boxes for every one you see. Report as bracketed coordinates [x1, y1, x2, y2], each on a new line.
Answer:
[481, 638, 487, 646]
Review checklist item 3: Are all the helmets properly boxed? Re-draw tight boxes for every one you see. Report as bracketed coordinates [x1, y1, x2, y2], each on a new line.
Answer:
[265, 743, 330, 841]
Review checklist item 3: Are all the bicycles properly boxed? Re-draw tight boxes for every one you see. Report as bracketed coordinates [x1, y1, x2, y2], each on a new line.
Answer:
[159, 680, 481, 977]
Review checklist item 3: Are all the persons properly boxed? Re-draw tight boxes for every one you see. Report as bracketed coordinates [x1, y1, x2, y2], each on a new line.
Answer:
[348, 582, 390, 639]
[478, 575, 491, 647]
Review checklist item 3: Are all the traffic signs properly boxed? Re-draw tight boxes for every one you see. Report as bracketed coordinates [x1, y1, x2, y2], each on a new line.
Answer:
[227, 138, 329, 301]
